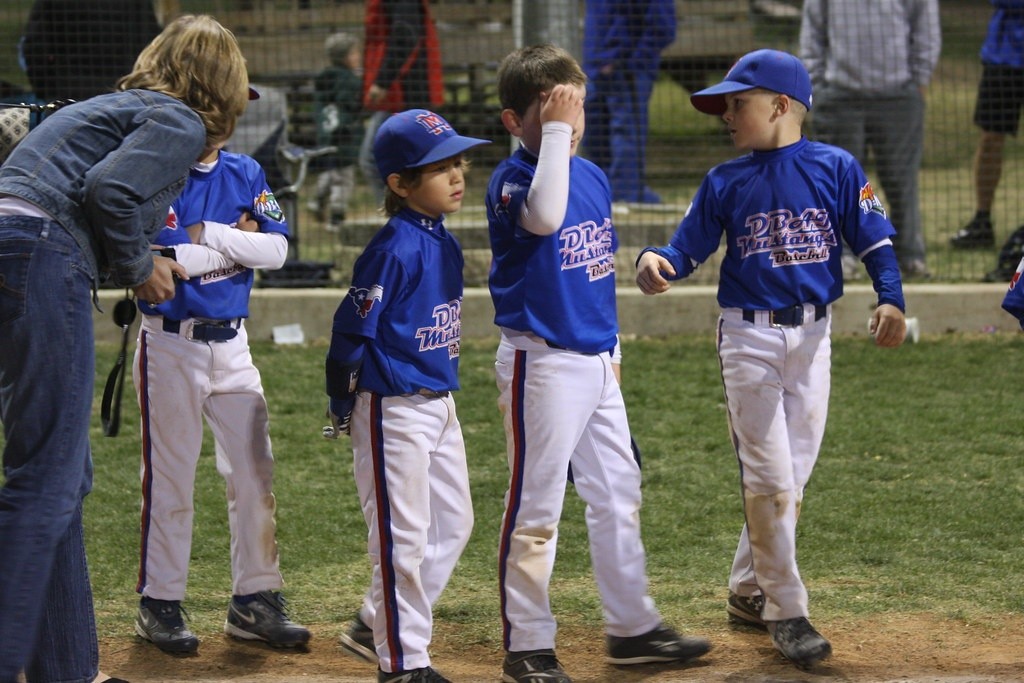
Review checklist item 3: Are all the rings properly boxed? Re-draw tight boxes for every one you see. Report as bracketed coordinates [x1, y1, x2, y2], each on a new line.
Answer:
[150, 303, 155, 307]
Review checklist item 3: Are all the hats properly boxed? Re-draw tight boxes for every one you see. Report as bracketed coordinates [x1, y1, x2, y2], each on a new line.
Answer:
[374, 109, 493, 176]
[248, 87, 260, 100]
[689, 49, 813, 117]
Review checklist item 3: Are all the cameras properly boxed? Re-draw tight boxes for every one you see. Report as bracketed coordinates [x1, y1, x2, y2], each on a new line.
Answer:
[150, 248, 181, 279]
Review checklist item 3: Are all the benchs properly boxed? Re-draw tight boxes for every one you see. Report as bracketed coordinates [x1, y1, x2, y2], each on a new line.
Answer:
[440, 59, 511, 167]
[253, 72, 376, 169]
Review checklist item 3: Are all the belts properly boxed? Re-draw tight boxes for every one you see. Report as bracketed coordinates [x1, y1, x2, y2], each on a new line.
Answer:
[742, 302, 827, 327]
[163, 316, 239, 341]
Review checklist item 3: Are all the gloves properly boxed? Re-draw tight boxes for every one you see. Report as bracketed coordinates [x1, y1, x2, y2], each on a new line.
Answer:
[322, 395, 352, 439]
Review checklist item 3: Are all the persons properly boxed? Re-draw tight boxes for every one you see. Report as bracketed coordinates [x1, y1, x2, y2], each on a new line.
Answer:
[581, 1, 680, 204]
[359, 0, 444, 221]
[22, 0, 166, 130]
[307, 30, 371, 227]
[130, 86, 313, 659]
[632, 48, 910, 664]
[485, 41, 714, 683]
[319, 112, 493, 683]
[796, 0, 941, 278]
[0, 14, 248, 683]
[947, 0, 1024, 249]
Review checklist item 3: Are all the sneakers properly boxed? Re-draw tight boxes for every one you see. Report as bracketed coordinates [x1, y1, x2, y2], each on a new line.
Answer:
[222, 588, 310, 648]
[502, 645, 571, 683]
[378, 659, 448, 682]
[726, 588, 768, 629]
[339, 615, 379, 664]
[605, 624, 712, 665]
[133, 592, 199, 652]
[767, 612, 832, 669]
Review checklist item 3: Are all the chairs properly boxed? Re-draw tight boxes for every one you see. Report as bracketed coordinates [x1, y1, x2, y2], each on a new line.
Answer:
[219, 81, 342, 269]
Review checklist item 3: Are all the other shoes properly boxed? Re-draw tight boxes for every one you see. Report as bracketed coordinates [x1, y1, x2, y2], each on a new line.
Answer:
[951, 217, 994, 247]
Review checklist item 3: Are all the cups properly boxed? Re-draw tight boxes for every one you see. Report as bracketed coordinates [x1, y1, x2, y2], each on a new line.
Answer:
[867, 316, 919, 346]
